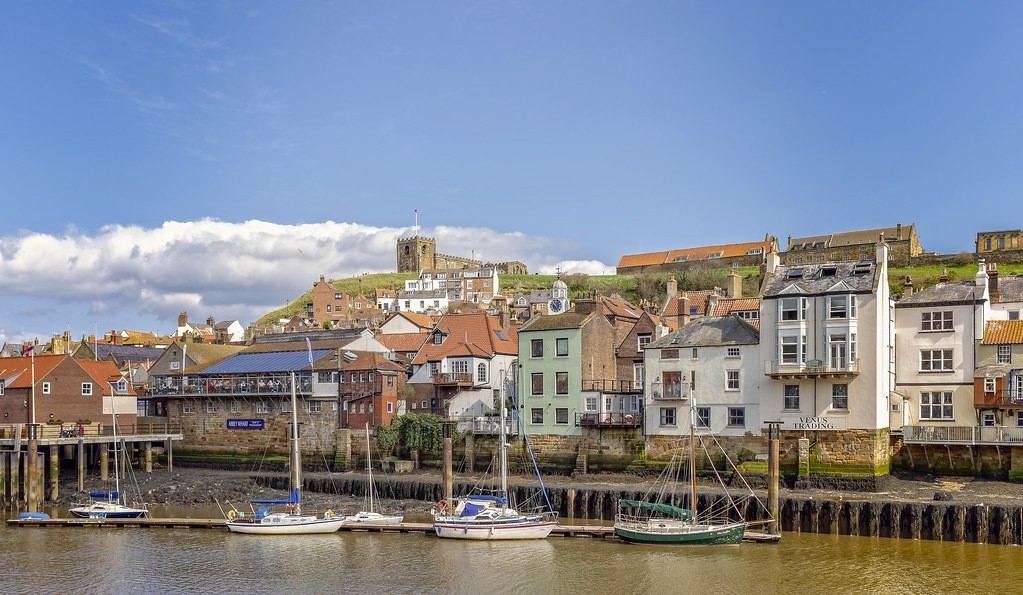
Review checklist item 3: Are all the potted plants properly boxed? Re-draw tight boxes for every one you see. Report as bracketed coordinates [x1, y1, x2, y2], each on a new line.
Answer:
[1004, 432, 1010, 441]
[323, 319, 333, 330]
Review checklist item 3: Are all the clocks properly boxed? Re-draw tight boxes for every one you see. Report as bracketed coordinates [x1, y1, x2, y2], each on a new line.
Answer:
[550, 299, 562, 313]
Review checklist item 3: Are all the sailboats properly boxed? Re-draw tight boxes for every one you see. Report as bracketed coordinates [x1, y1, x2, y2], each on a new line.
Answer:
[218, 370, 348, 537]
[329, 420, 410, 525]
[611, 381, 783, 552]
[429, 365, 566, 540]
[63, 386, 152, 519]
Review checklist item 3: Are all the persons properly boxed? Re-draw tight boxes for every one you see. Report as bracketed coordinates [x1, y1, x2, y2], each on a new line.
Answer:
[79, 425, 84, 437]
[144, 377, 310, 395]
[59, 428, 70, 438]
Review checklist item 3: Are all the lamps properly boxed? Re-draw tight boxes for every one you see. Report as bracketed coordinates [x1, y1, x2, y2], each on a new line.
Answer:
[656, 376, 659, 381]
[116, 414, 119, 418]
[683, 375, 686, 381]
[49, 414, 54, 418]
[5, 412, 9, 417]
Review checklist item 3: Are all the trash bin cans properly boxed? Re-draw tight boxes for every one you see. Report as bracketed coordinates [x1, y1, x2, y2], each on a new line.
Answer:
[28, 426, 37, 439]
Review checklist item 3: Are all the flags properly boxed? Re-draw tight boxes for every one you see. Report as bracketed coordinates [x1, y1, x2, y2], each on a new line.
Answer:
[25, 340, 34, 357]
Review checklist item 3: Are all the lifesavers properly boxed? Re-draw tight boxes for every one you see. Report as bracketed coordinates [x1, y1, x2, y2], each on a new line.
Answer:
[437, 499, 449, 511]
[623, 415, 634, 424]
[228, 510, 235, 519]
[604, 418, 614, 424]
[286, 498, 296, 508]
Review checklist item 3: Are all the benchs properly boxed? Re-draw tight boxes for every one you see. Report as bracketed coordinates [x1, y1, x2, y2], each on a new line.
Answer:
[801, 359, 823, 372]
